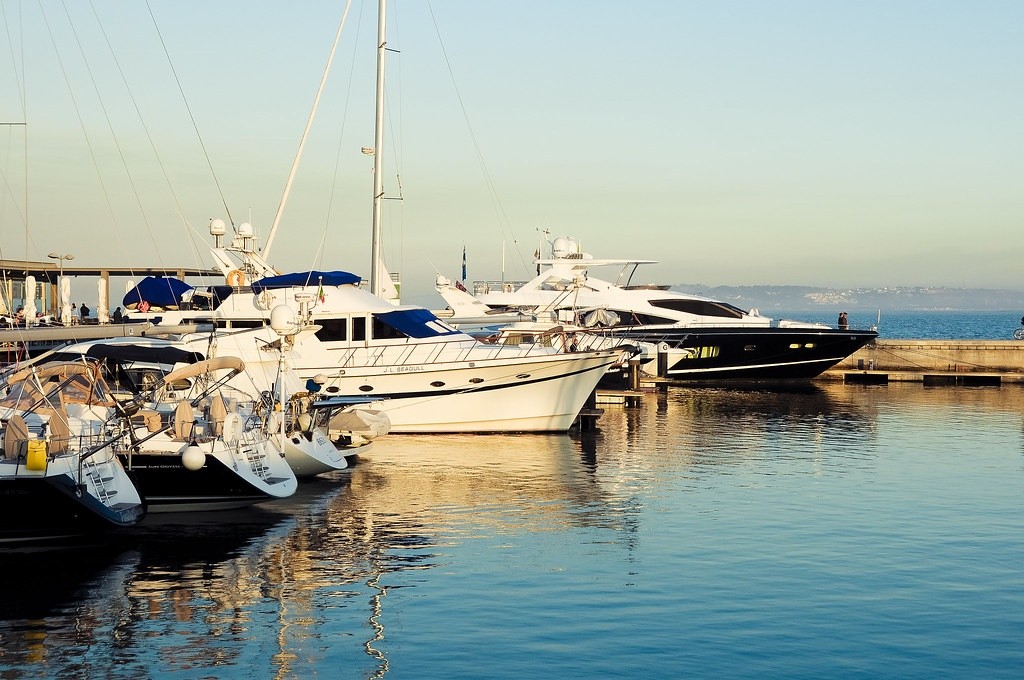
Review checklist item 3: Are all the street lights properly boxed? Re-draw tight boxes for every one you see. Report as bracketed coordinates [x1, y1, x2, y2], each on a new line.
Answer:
[48, 252, 75, 324]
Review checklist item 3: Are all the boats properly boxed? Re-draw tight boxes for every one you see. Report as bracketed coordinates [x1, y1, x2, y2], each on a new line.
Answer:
[474, 236, 879, 383]
[433, 275, 690, 390]
[0, 336, 392, 587]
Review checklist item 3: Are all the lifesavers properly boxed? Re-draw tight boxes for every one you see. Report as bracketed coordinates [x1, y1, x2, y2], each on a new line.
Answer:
[225, 270, 245, 288]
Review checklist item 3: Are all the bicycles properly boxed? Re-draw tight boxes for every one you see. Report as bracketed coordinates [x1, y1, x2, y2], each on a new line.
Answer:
[1013, 322, 1024, 339]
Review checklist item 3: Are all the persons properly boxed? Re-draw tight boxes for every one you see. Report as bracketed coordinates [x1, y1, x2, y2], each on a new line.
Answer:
[71, 303, 78, 322]
[113, 307, 124, 324]
[36, 307, 62, 322]
[570, 339, 578, 352]
[838, 312, 848, 330]
[80, 303, 90, 325]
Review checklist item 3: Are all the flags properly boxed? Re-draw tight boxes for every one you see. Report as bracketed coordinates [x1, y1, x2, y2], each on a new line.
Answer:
[462, 248, 467, 281]
[319, 287, 325, 305]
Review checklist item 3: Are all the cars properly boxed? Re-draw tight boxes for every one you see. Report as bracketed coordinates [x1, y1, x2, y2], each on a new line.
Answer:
[0, 314, 20, 324]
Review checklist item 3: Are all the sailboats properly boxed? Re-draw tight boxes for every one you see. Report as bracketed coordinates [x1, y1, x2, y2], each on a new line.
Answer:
[178, 0, 627, 435]
